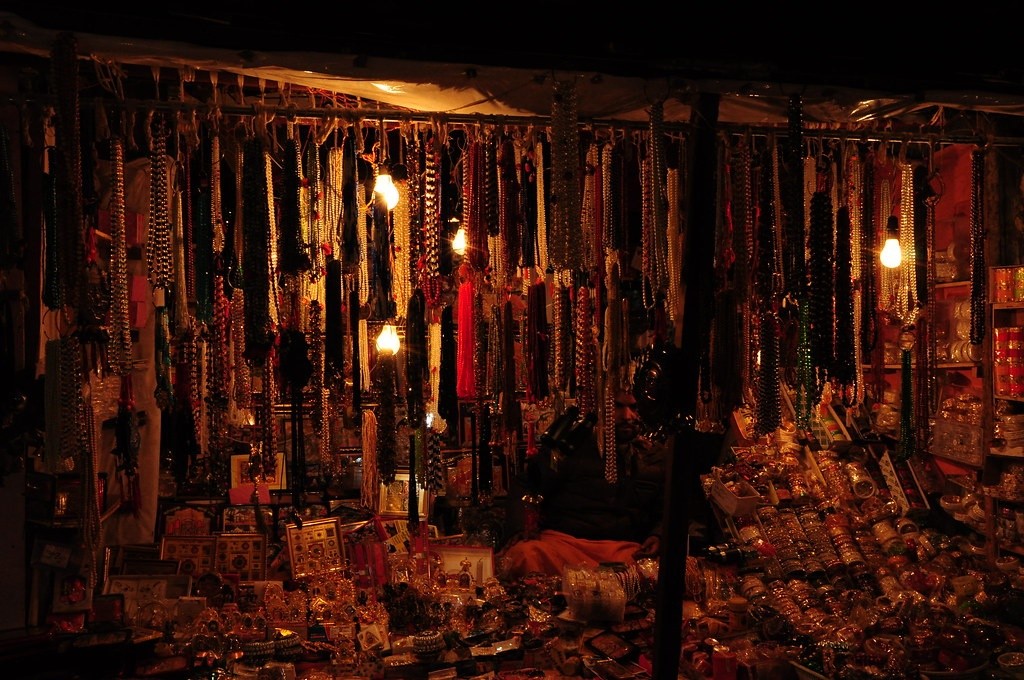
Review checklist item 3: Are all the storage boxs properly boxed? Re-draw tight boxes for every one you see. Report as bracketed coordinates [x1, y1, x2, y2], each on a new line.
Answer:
[711, 476, 761, 515]
[808, 404, 852, 451]
[878, 450, 931, 519]
[989, 263, 1024, 304]
[732, 413, 768, 446]
[432, 542, 495, 578]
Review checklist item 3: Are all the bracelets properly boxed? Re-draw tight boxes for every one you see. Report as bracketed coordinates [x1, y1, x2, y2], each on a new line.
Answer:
[699, 300, 1024, 680]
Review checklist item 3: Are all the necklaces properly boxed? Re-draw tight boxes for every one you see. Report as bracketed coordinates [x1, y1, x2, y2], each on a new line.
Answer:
[42, 108, 987, 483]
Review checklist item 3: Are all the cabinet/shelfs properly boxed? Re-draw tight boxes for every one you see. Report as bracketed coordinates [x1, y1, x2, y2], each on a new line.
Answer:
[862, 279, 988, 473]
[989, 302, 1024, 558]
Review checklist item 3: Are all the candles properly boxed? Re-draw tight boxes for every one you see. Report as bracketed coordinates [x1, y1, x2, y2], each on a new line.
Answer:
[477, 558, 483, 583]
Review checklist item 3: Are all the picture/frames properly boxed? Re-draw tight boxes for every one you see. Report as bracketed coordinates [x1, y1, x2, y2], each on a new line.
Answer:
[36, 403, 430, 619]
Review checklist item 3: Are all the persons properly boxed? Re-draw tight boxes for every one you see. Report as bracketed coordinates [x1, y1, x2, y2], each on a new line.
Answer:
[519, 386, 667, 558]
